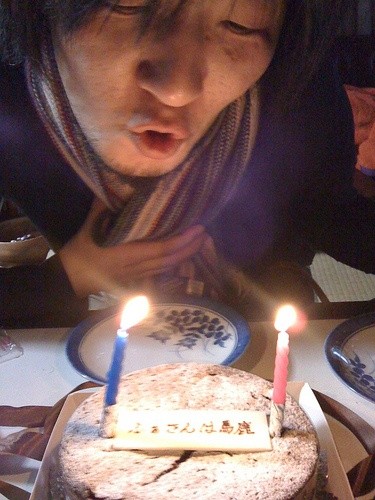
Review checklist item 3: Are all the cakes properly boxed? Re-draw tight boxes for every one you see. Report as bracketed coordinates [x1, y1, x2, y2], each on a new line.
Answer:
[60, 361, 321, 500]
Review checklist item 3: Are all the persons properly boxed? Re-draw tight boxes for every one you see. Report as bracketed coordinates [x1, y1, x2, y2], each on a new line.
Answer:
[0, 0, 355, 331]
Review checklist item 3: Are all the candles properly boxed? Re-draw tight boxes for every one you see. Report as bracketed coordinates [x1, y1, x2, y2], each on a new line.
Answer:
[268, 304, 298, 438]
[98, 294, 150, 438]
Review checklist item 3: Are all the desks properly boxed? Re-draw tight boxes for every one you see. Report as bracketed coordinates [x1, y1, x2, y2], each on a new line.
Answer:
[0, 320, 375, 500]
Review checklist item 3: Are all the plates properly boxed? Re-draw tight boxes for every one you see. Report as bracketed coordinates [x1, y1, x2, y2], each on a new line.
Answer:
[66, 296, 252, 385]
[29, 380, 353, 496]
[325, 312, 375, 405]
